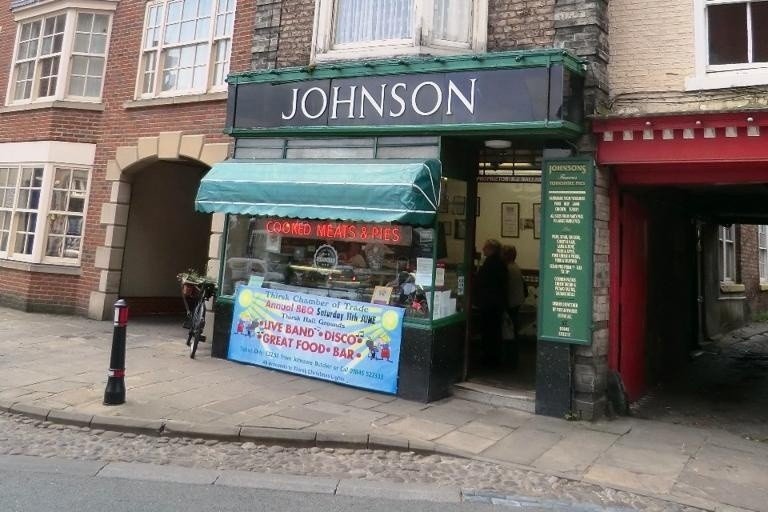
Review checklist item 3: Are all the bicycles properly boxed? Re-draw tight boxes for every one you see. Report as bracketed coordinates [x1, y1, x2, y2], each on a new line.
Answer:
[176, 269, 221, 358]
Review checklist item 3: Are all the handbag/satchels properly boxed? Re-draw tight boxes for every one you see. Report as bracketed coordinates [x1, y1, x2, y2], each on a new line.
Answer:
[501, 311, 515, 340]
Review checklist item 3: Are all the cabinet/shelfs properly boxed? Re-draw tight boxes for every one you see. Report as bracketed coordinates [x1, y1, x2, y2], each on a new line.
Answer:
[208, 202, 465, 404]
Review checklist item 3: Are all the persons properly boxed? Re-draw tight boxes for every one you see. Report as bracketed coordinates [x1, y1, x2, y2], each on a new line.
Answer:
[471, 237, 509, 372]
[500, 244, 527, 371]
[338, 241, 368, 268]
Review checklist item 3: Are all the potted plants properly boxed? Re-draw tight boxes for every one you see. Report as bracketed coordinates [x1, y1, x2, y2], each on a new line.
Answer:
[179, 270, 202, 296]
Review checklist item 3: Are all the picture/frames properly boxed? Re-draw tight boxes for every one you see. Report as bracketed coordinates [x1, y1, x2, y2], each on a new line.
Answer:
[501, 202, 541, 239]
